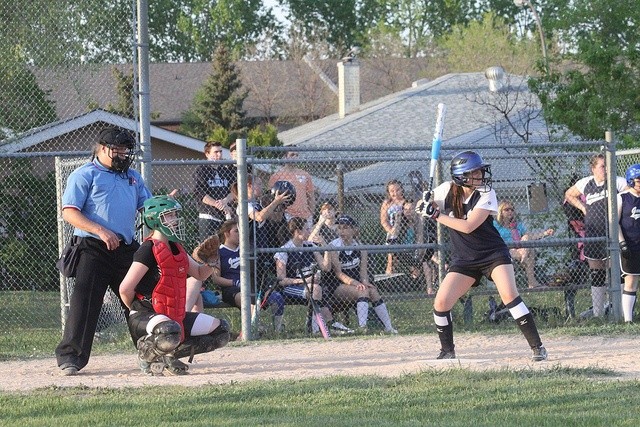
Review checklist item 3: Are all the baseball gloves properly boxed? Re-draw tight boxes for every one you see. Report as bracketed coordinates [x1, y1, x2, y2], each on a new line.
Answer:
[191, 233, 220, 267]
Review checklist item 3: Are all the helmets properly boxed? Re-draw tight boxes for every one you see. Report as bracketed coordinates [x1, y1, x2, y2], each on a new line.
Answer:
[625, 163, 640, 188]
[142, 196, 187, 242]
[96, 127, 137, 180]
[449, 150, 492, 194]
[272, 180, 296, 207]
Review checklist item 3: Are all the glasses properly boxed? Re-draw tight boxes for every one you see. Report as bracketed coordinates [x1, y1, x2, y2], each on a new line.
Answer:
[501, 207, 515, 212]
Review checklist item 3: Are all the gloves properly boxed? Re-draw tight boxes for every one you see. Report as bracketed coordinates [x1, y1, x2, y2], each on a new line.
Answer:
[422, 191, 433, 203]
[415, 199, 440, 220]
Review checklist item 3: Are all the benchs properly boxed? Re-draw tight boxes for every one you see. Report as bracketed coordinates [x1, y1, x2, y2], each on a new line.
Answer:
[205, 297, 360, 335]
[456, 281, 590, 330]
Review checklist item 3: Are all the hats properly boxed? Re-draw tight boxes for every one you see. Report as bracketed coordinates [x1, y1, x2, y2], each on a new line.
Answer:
[335, 216, 360, 228]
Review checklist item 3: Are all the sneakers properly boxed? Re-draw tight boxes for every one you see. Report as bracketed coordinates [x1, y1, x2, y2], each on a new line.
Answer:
[163, 355, 190, 376]
[136, 336, 164, 377]
[533, 347, 548, 362]
[58, 366, 78, 376]
[435, 350, 456, 360]
[357, 327, 368, 335]
[329, 321, 356, 335]
[383, 328, 398, 335]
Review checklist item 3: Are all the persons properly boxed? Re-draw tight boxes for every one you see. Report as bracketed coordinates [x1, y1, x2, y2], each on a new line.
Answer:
[382, 180, 421, 279]
[119, 194, 230, 375]
[329, 215, 399, 334]
[231, 173, 292, 281]
[492, 199, 554, 289]
[276, 216, 356, 337]
[56, 127, 157, 376]
[193, 140, 236, 248]
[415, 150, 548, 361]
[390, 198, 424, 293]
[560, 172, 613, 321]
[616, 163, 640, 323]
[305, 198, 340, 248]
[223, 141, 254, 179]
[219, 219, 285, 341]
[266, 143, 317, 235]
[565, 153, 628, 323]
[411, 169, 452, 295]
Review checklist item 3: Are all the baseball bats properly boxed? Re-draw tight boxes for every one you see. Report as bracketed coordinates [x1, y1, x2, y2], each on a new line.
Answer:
[305, 263, 317, 336]
[250, 261, 270, 325]
[428, 102, 448, 202]
[235, 276, 281, 341]
[297, 264, 331, 341]
